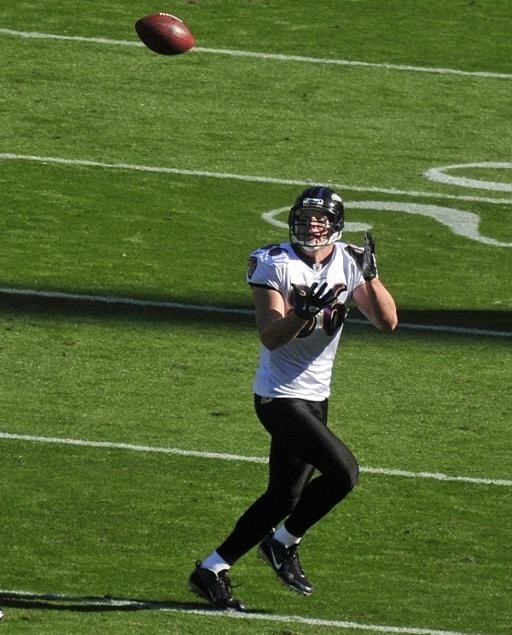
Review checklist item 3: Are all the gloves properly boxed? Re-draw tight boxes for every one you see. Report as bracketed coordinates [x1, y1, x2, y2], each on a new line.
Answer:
[289, 282, 337, 320]
[344, 231, 378, 281]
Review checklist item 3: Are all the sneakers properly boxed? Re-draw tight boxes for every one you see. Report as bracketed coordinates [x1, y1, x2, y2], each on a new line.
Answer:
[257, 530, 314, 596]
[188, 560, 245, 612]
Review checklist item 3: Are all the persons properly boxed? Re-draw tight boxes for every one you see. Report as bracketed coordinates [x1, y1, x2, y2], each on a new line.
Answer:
[189, 187, 398, 609]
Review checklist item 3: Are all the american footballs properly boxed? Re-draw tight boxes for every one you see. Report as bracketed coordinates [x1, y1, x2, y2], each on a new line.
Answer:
[135, 13, 197, 55]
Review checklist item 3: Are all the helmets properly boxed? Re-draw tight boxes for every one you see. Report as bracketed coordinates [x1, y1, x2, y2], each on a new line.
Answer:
[288, 186, 345, 247]
